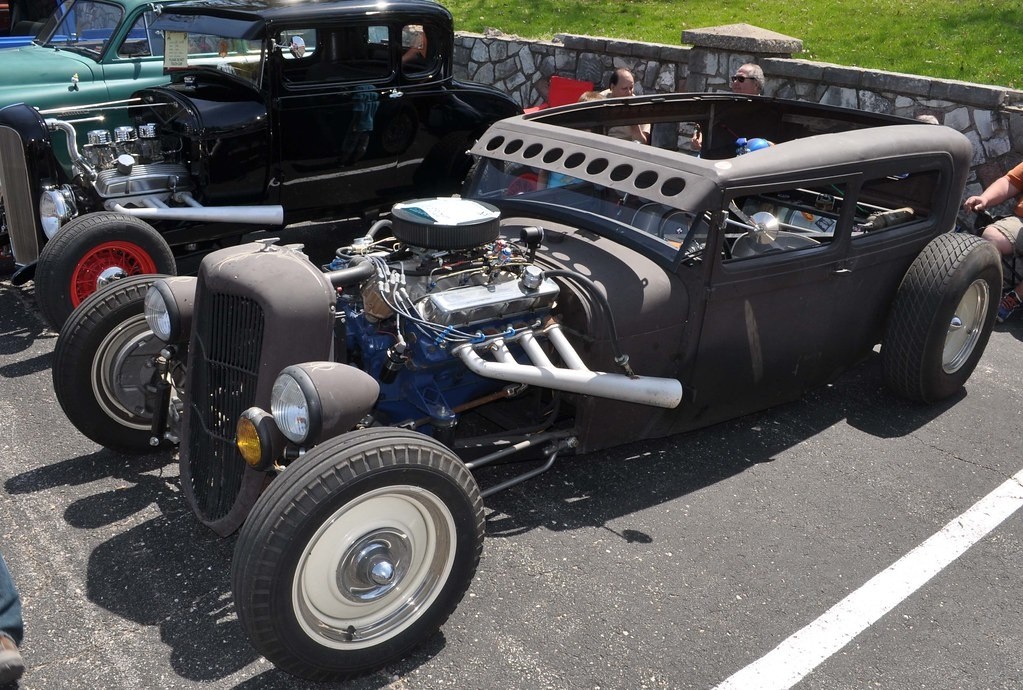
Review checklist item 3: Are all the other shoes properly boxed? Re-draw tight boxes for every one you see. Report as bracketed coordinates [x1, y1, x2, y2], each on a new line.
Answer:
[0, 635, 25, 685]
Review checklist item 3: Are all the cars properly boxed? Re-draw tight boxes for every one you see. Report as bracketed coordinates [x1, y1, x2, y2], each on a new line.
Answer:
[0, 0, 318, 186]
[0, 0, 527, 336]
[52, 92, 1003, 680]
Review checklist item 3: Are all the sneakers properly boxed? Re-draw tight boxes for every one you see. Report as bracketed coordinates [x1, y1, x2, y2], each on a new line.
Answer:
[996, 291, 1022, 323]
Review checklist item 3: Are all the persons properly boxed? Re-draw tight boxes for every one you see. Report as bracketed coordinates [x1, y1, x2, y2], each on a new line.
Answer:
[0, 554, 25, 690]
[962, 161, 1023, 326]
[691, 62, 765, 170]
[536, 69, 651, 201]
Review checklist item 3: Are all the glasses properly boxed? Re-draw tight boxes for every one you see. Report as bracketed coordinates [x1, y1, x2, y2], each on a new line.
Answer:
[731, 75, 756, 83]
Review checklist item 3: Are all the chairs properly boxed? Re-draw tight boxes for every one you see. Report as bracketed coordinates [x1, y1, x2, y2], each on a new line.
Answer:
[971, 207, 1023, 292]
[523, 76, 594, 115]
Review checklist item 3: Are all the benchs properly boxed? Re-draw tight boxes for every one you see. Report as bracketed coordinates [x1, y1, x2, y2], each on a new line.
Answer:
[306, 59, 423, 84]
